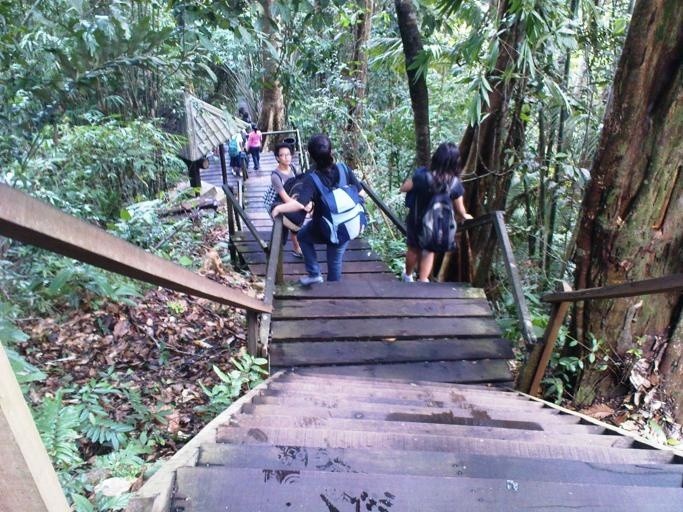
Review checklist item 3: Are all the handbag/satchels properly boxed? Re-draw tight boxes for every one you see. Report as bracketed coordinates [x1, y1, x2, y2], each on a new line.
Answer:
[264, 185, 278, 206]
[275, 173, 309, 233]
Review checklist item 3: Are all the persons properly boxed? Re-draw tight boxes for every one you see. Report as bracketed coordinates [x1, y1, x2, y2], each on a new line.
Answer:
[272, 136, 365, 285]
[207, 107, 262, 180]
[400, 139, 477, 283]
[259, 142, 315, 259]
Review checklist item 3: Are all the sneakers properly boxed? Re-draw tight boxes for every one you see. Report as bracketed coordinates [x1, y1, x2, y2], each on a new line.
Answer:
[231, 168, 243, 177]
[292, 249, 304, 258]
[300, 274, 324, 286]
[254, 166, 259, 170]
[402, 269, 429, 283]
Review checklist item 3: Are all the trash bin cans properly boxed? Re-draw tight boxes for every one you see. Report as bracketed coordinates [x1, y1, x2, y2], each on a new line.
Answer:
[283, 137, 296, 155]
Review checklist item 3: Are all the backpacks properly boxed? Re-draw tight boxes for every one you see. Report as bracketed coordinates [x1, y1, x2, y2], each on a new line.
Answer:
[418, 170, 458, 253]
[309, 163, 367, 244]
[228, 135, 239, 158]
